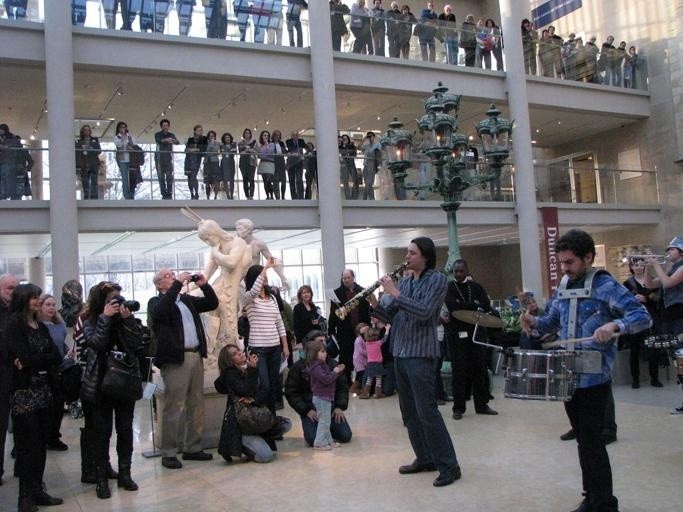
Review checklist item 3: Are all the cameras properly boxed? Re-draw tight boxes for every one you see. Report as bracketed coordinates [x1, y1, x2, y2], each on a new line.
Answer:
[367, 135, 371, 137]
[271, 257, 281, 265]
[111, 295, 140, 312]
[249, 349, 260, 361]
[187, 274, 199, 282]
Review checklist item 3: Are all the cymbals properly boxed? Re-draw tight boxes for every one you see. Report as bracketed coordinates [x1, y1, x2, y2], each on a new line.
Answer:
[451, 310, 506, 329]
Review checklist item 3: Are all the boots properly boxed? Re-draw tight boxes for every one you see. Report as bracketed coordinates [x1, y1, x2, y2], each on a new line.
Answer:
[81, 427, 137, 497]
[18, 479, 63, 512]
[351, 381, 382, 399]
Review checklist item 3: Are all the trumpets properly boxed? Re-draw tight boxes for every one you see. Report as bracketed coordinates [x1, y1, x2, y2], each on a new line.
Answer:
[611, 249, 669, 266]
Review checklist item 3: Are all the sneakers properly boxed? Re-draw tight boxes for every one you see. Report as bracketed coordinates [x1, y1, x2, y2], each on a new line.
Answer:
[453, 411, 461, 419]
[560, 429, 575, 440]
[479, 408, 497, 415]
[651, 377, 663, 387]
[632, 379, 640, 389]
[183, 451, 213, 460]
[433, 465, 461, 486]
[47, 440, 69, 451]
[399, 459, 436, 473]
[437, 398, 445, 405]
[314, 442, 340, 450]
[675, 404, 683, 413]
[162, 457, 182, 468]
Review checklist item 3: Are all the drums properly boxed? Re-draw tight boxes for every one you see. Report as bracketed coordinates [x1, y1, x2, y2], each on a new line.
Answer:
[503, 348, 574, 402]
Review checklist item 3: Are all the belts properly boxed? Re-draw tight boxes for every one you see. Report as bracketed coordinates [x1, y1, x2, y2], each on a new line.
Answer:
[185, 345, 199, 352]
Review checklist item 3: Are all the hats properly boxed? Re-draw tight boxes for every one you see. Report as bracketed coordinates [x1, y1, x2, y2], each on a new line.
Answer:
[666, 236, 682, 252]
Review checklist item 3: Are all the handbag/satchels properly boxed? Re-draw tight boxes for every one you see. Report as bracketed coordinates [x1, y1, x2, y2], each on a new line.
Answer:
[228, 393, 277, 433]
[249, 154, 257, 167]
[101, 342, 144, 400]
[57, 356, 82, 403]
